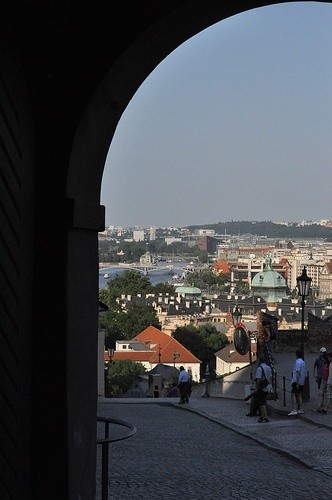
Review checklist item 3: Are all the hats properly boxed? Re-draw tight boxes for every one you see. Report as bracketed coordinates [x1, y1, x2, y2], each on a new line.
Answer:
[320, 347, 326, 352]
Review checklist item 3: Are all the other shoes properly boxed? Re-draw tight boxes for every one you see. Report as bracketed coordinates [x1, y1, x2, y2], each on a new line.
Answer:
[244, 396, 251, 401]
[297, 410, 304, 413]
[246, 413, 257, 416]
[258, 417, 269, 423]
[288, 411, 298, 415]
[178, 401, 185, 404]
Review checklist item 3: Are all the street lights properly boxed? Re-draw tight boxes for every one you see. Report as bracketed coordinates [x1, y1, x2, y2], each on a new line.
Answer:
[107, 345, 115, 363]
[172, 350, 178, 368]
[295, 265, 313, 361]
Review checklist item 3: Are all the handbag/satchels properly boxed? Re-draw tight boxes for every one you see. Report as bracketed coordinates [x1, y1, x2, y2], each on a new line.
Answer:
[259, 379, 269, 390]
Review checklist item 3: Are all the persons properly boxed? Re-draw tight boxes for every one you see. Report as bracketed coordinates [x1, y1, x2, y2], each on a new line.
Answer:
[288, 350, 307, 415]
[313, 347, 332, 415]
[245, 378, 260, 417]
[177, 366, 189, 405]
[256, 356, 272, 423]
